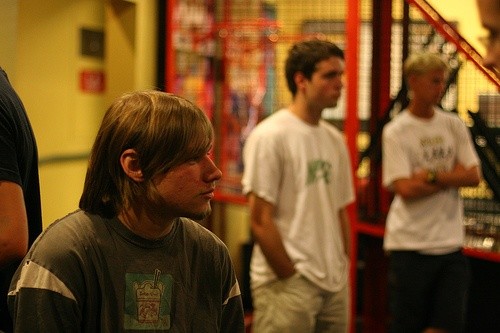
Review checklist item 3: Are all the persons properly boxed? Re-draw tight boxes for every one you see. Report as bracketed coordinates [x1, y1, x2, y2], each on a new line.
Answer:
[1, 66, 44, 333]
[377, 54, 483, 333]
[241, 39, 359, 333]
[7, 89, 245, 333]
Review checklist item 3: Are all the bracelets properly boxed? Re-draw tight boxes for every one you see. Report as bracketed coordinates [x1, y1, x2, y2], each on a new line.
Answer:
[428, 171, 437, 183]
